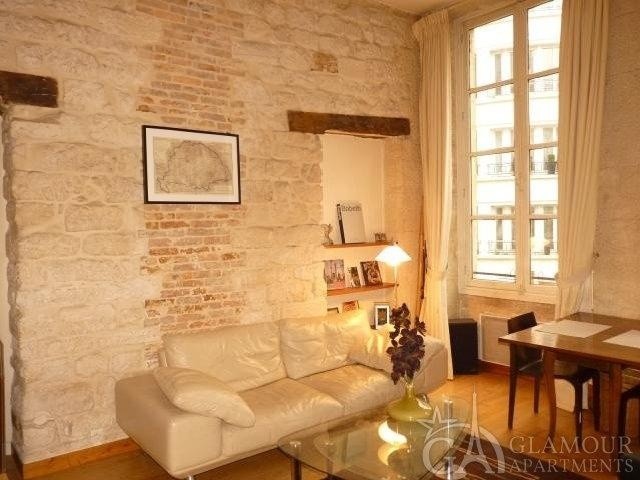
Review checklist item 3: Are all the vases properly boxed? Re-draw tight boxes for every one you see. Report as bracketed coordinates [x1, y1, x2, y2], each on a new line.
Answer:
[386, 383, 434, 423]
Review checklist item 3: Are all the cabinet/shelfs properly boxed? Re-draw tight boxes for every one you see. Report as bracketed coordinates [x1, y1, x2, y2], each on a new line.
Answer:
[324, 241, 397, 330]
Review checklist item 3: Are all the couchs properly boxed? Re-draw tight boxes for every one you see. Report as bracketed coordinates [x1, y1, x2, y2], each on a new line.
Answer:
[114, 304, 450, 480]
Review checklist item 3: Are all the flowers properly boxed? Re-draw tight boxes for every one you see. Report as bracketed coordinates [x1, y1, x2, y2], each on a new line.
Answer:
[385, 302, 427, 387]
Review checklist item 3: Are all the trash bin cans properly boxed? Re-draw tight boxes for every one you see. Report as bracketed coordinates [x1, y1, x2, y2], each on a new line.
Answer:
[600, 368, 640, 437]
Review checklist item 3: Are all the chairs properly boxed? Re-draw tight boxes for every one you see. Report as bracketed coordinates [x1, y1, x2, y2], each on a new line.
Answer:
[507, 311, 599, 449]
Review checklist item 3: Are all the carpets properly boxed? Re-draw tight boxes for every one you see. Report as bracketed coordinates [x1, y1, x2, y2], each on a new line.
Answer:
[319, 428, 594, 480]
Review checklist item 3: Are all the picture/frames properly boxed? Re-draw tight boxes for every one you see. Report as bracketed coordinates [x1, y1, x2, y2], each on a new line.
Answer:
[373, 303, 391, 330]
[140, 123, 241, 204]
[326, 307, 340, 314]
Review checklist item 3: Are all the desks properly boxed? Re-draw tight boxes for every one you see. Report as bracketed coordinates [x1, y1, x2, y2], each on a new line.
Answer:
[499, 311, 639, 474]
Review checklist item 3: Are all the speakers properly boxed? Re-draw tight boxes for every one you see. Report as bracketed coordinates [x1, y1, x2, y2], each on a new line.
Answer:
[448, 318, 478, 374]
